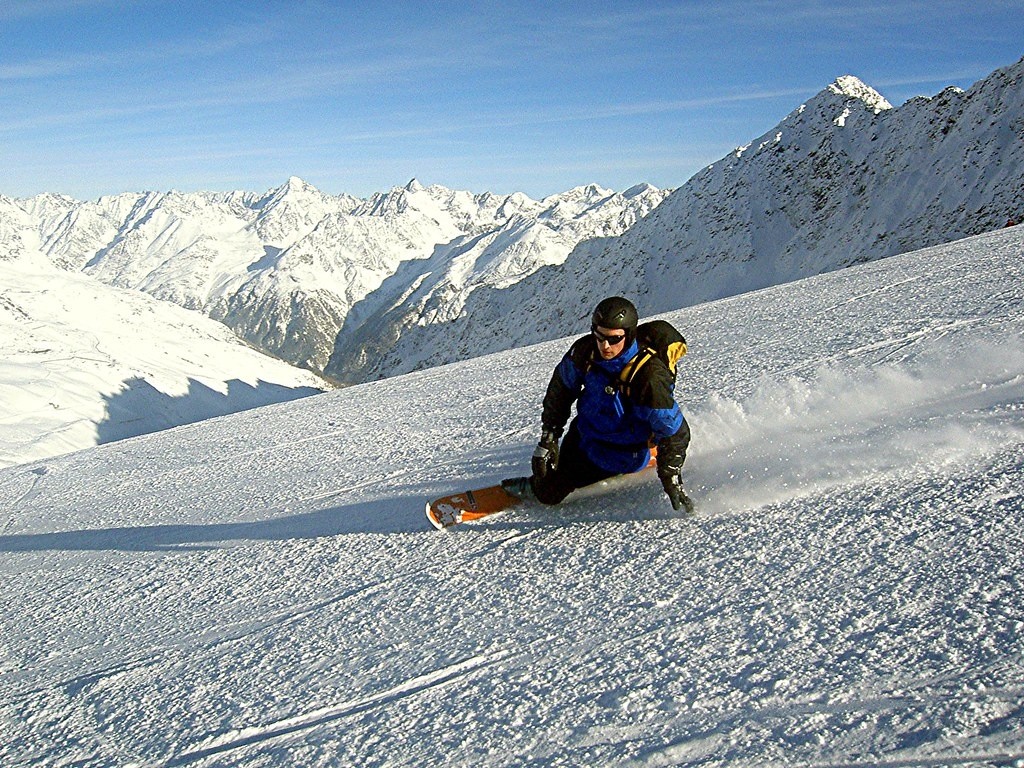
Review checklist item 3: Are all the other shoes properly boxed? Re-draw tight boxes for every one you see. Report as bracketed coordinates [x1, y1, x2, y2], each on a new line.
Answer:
[501, 477, 535, 501]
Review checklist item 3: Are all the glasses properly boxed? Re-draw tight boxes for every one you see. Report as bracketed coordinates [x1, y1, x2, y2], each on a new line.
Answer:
[593, 329, 627, 345]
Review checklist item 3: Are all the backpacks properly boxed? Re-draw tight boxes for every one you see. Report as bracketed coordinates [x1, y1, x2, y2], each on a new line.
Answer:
[585, 320, 688, 448]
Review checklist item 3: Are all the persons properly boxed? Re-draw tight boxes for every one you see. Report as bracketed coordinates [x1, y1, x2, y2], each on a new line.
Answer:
[499, 296, 694, 514]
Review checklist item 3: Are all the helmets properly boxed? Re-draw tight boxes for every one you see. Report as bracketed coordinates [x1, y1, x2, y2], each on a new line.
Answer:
[589, 296, 638, 347]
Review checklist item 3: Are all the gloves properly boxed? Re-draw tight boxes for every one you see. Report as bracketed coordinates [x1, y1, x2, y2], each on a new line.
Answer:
[531, 430, 559, 480]
[665, 476, 693, 512]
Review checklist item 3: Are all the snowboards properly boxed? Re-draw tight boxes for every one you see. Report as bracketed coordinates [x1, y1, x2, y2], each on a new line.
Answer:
[423, 446, 659, 531]
